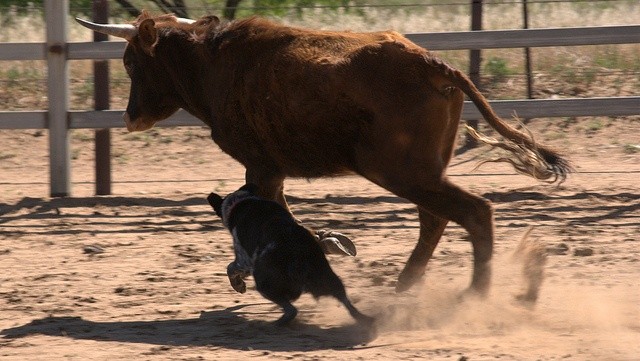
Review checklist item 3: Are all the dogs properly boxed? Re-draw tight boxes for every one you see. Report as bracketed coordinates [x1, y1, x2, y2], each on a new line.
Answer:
[207, 183, 379, 334]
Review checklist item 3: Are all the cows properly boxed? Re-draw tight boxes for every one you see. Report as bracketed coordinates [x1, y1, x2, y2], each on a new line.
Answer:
[73, 9, 578, 309]
